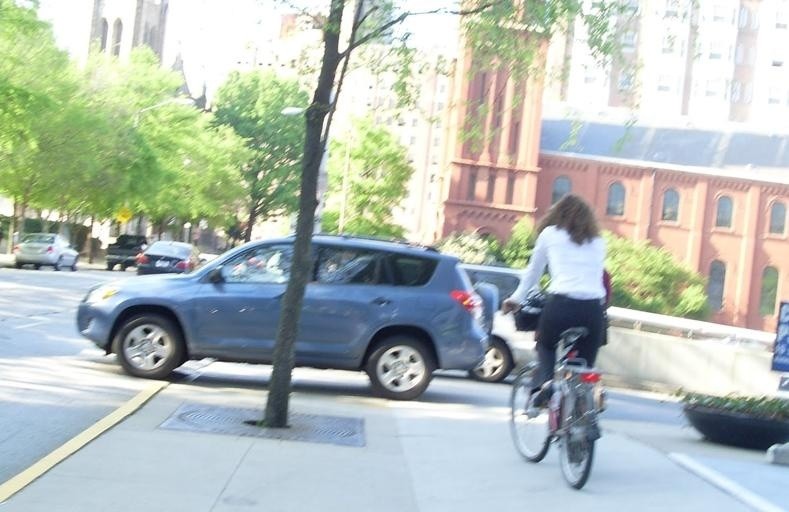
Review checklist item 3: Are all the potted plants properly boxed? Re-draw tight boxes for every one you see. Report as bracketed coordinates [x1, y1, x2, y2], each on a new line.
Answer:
[664, 387, 788, 451]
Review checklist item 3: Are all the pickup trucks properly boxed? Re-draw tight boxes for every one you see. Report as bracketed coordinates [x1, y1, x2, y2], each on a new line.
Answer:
[106, 232, 151, 273]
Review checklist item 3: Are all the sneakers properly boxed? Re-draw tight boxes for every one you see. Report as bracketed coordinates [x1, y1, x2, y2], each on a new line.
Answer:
[526, 381, 553, 419]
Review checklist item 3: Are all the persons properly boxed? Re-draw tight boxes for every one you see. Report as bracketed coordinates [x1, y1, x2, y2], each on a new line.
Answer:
[500, 193, 610, 420]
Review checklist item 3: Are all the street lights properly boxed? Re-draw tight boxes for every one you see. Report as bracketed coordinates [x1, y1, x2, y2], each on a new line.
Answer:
[279, 106, 354, 236]
[135, 97, 197, 127]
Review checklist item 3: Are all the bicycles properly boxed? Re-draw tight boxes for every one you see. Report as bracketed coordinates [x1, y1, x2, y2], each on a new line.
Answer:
[497, 292, 610, 492]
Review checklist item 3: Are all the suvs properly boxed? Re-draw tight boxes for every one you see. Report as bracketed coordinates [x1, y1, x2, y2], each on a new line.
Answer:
[75, 233, 498, 401]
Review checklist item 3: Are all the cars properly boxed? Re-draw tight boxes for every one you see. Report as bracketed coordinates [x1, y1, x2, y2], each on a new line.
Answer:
[12, 231, 81, 271]
[453, 259, 544, 387]
[133, 239, 207, 278]
